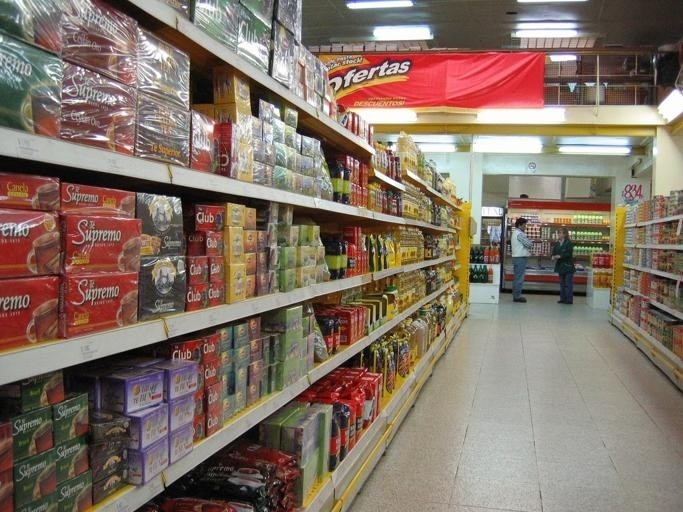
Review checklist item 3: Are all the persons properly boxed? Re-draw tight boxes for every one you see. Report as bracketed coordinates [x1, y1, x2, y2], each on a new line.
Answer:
[511, 218, 534, 302]
[551, 227, 577, 304]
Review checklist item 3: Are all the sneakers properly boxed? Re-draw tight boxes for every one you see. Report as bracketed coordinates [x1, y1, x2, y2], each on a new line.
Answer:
[557, 300, 573, 304]
[513, 296, 527, 303]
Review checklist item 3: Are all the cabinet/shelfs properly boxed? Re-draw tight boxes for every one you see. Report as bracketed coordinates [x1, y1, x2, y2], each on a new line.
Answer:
[503, 198, 610, 292]
[469, 242, 504, 305]
[585, 252, 612, 310]
[0, 0, 472, 512]
[608, 191, 683, 394]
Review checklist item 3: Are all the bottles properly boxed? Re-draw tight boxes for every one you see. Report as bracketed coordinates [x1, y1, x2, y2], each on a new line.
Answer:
[469, 246, 501, 283]
[326, 131, 446, 357]
